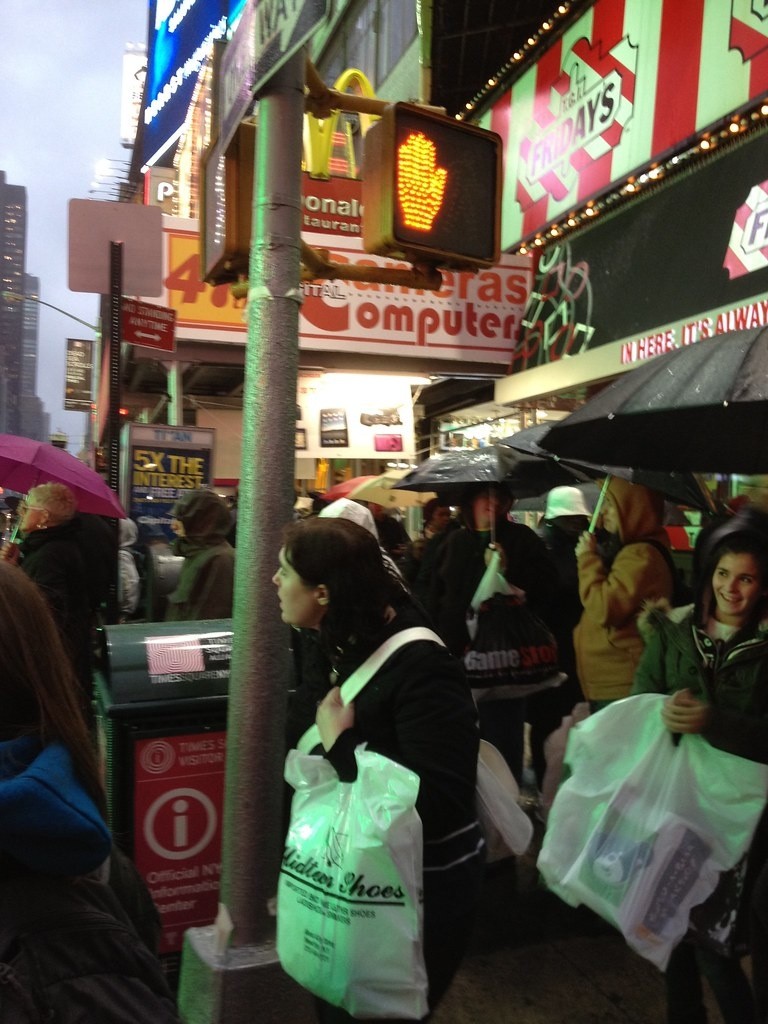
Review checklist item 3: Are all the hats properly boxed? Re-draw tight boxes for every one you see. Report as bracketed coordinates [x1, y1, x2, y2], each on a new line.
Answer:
[544, 486, 592, 520]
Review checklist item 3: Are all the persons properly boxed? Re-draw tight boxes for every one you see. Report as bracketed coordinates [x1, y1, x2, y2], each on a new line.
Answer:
[0, 482, 160, 760]
[304, 477, 767, 1024]
[0, 558, 161, 1024]
[272, 517, 482, 1024]
[166, 490, 238, 622]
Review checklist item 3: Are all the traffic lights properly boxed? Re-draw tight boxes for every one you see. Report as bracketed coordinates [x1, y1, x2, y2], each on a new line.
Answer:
[362, 102, 504, 270]
[199, 122, 256, 286]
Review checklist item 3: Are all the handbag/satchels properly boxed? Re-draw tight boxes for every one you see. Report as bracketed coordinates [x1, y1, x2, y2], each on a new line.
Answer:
[472, 739, 534, 865]
[276, 742, 429, 1020]
[536, 693, 768, 972]
[455, 552, 568, 710]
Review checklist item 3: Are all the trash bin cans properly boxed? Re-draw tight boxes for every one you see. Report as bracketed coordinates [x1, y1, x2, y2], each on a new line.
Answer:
[93, 618, 230, 952]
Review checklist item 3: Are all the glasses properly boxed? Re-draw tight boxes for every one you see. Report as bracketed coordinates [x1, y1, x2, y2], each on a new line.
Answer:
[22, 503, 44, 511]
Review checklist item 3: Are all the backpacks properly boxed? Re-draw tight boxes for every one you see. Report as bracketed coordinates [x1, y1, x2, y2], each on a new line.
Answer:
[636, 538, 695, 607]
[0, 871, 183, 1024]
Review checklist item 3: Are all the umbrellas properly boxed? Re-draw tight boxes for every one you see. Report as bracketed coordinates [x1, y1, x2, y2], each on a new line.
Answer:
[390, 446, 574, 542]
[499, 421, 719, 533]
[536, 325, 768, 476]
[320, 476, 377, 506]
[1, 432, 127, 545]
[346, 470, 441, 510]
